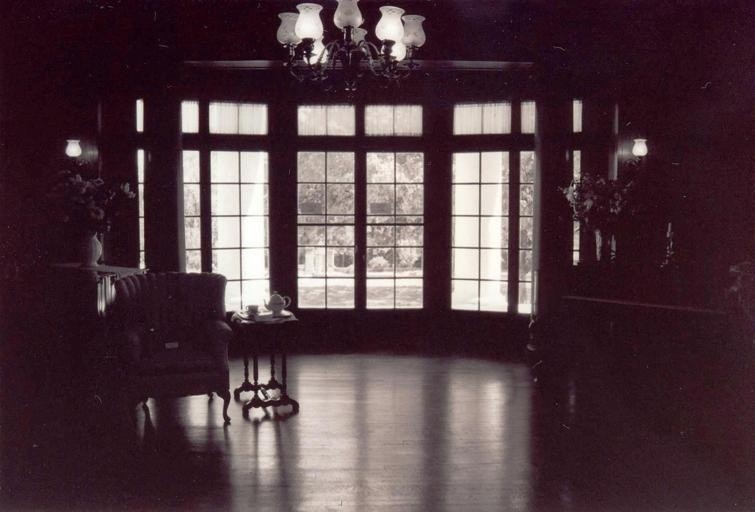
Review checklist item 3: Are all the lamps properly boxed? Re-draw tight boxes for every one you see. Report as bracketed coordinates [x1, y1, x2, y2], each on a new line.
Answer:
[277, 0, 426, 99]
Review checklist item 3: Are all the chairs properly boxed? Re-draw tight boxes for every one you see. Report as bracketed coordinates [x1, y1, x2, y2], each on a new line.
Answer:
[114, 272, 233, 423]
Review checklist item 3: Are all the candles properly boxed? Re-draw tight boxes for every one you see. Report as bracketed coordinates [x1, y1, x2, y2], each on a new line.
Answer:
[668, 221, 672, 232]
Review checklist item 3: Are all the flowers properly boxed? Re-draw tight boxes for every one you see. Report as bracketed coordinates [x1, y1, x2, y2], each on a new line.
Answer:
[559, 173, 642, 226]
[44, 161, 136, 234]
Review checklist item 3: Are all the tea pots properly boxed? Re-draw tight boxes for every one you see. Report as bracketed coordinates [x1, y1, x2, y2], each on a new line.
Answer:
[263, 290, 292, 318]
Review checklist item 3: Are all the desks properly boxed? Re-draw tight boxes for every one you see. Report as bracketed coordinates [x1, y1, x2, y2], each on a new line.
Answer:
[235, 315, 300, 417]
[29, 262, 148, 320]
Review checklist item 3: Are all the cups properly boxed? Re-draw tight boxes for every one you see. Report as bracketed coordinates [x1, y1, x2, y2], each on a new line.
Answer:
[245, 305, 258, 313]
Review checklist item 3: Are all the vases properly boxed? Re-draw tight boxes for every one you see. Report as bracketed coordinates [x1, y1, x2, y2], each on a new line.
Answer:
[81, 233, 105, 268]
[595, 229, 616, 277]
